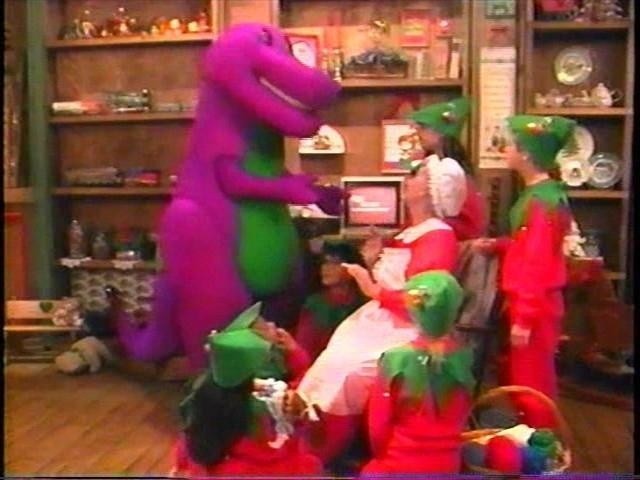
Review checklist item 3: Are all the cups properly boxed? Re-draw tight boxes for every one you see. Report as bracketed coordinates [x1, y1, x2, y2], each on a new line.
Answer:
[534, 91, 591, 109]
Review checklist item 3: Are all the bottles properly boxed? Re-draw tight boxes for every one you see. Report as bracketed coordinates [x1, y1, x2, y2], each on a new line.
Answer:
[582, 229, 600, 258]
[68, 220, 111, 261]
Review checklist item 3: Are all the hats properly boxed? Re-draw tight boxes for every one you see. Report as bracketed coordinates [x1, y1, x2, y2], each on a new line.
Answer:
[425, 154, 468, 219]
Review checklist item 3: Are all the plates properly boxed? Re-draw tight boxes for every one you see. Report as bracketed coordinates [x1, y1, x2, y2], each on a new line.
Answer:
[552, 46, 593, 86]
[555, 123, 621, 188]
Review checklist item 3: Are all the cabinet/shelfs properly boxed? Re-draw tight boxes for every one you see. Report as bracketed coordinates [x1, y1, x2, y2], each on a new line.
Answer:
[51, 0, 223, 333]
[514, 0, 635, 346]
[272, 0, 471, 248]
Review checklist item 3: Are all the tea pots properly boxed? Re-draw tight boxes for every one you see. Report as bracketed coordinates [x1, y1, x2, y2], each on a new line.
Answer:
[582, 82, 622, 109]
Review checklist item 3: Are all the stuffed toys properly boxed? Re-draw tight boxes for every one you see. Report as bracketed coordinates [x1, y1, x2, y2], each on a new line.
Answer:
[111, 20, 354, 383]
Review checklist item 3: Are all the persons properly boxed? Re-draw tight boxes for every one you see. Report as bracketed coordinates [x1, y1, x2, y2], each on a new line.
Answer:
[169, 103, 575, 480]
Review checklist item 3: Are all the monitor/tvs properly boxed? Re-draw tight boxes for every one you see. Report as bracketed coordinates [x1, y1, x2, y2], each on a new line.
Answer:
[340, 177, 405, 238]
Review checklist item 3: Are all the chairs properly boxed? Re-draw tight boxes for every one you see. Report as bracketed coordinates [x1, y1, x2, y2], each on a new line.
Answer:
[347, 238, 500, 427]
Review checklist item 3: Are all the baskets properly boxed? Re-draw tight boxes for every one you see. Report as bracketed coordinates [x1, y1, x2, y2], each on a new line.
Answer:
[462, 383, 574, 478]
